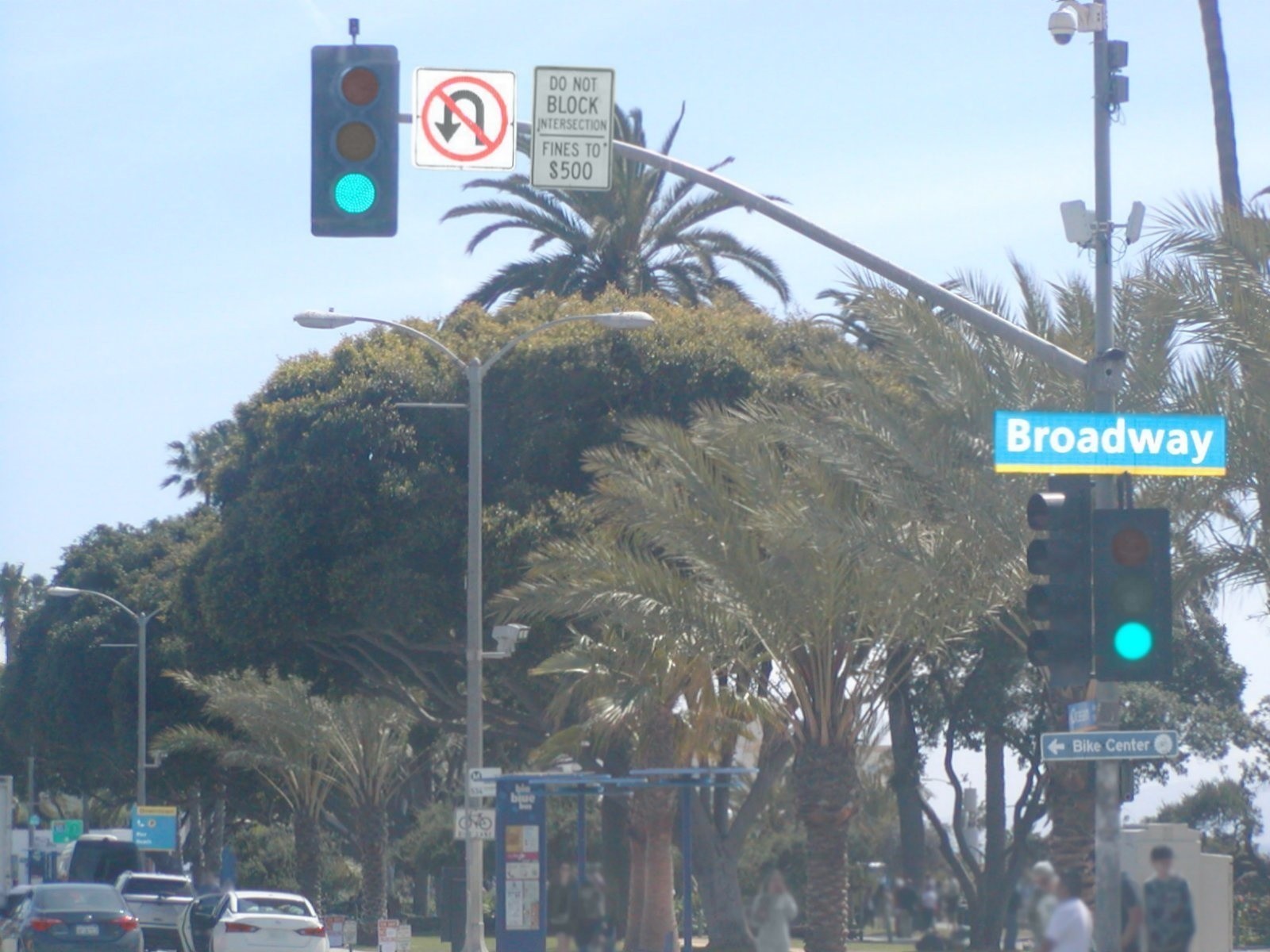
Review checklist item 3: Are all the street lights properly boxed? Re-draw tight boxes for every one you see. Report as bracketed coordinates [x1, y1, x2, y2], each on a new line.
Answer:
[289, 309, 664, 952]
[36, 582, 182, 807]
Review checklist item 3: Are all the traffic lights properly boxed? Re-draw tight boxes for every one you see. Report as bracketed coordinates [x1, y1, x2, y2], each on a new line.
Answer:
[1091, 506, 1179, 684]
[1025, 466, 1092, 681]
[307, 43, 399, 243]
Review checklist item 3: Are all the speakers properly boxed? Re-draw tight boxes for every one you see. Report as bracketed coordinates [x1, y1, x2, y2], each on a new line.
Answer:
[1125, 201, 1145, 242]
[1061, 200, 1091, 243]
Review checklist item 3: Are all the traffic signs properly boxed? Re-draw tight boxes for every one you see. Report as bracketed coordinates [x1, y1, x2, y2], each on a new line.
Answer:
[1041, 730, 1181, 764]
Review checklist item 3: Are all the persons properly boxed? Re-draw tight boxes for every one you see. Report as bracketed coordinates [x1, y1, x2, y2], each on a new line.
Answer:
[1028, 845, 1196, 952]
[890, 872, 962, 937]
[748, 868, 799, 952]
[547, 863, 578, 952]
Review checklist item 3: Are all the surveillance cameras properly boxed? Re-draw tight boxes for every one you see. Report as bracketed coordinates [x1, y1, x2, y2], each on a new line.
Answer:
[1049, 13, 1076, 45]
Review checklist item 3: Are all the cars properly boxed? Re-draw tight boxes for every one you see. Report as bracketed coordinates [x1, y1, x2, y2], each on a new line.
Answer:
[0, 832, 200, 952]
[176, 888, 330, 952]
